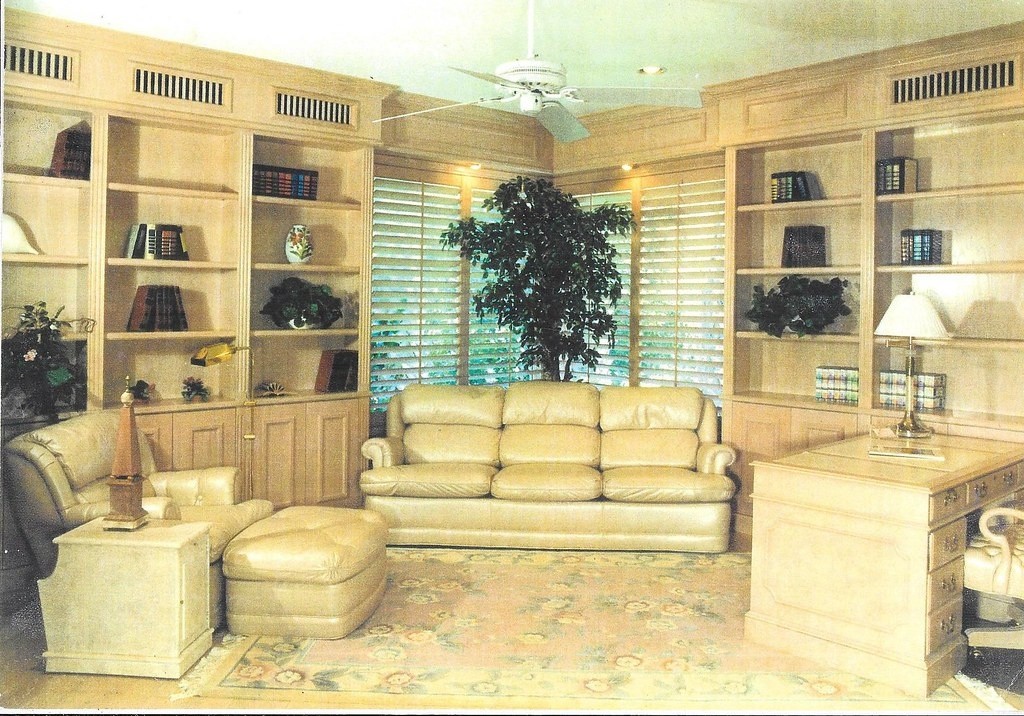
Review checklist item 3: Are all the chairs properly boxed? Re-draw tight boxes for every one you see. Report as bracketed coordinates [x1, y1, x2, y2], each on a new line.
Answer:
[963, 507, 1024, 663]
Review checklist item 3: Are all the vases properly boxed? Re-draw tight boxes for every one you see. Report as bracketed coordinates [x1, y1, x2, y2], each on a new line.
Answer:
[1, 415, 58, 567]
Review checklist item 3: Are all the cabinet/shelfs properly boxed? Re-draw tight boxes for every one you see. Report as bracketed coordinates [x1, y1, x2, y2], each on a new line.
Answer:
[721, 102, 1023, 536]
[747, 433, 1024, 697]
[0, 81, 368, 512]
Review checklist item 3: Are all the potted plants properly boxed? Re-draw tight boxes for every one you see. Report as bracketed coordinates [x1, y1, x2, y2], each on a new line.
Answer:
[259, 277, 345, 332]
[745, 273, 853, 339]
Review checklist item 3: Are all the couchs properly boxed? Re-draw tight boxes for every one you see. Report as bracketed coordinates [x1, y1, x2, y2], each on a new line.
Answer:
[3, 409, 272, 635]
[358, 383, 737, 556]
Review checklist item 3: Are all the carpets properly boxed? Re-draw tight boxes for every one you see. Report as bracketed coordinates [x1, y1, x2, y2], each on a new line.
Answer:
[170, 548, 1021, 716]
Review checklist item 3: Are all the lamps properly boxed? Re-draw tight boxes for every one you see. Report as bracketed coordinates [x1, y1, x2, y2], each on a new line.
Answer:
[872, 290, 950, 439]
[190, 341, 255, 502]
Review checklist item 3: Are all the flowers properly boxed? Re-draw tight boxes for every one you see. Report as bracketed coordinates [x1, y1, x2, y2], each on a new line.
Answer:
[0, 299, 80, 418]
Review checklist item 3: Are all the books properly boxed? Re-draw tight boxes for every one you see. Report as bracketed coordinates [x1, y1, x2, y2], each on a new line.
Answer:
[253, 164, 318, 200]
[770, 172, 821, 203]
[879, 369, 946, 413]
[121, 224, 189, 261]
[901, 230, 942, 265]
[816, 367, 858, 403]
[875, 156, 918, 195]
[49, 129, 91, 181]
[779, 225, 827, 268]
[868, 445, 944, 460]
[315, 349, 358, 391]
[126, 285, 189, 332]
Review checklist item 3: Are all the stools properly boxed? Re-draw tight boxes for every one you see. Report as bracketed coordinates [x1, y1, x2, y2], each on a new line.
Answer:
[222, 503, 387, 642]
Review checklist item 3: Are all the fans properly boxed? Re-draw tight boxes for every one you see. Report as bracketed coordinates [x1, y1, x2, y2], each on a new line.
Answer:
[375, 2, 704, 147]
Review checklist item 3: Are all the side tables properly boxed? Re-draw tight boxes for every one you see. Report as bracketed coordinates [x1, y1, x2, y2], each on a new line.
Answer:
[39, 516, 214, 681]
